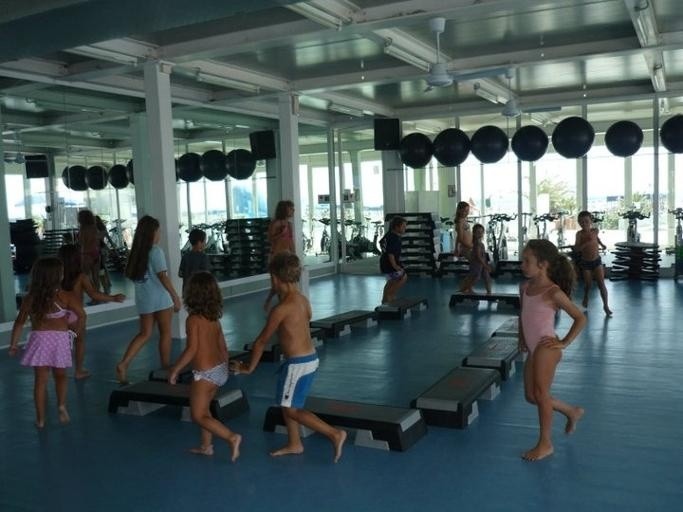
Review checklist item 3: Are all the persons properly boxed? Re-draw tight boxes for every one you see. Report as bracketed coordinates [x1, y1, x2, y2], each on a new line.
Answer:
[379, 216, 408, 303]
[116, 216, 181, 384]
[454, 201, 474, 291]
[59, 244, 126, 380]
[461, 224, 492, 293]
[574, 211, 612, 316]
[177, 229, 209, 299]
[519, 238, 588, 461]
[263, 200, 294, 314]
[168, 273, 242, 464]
[9, 257, 87, 429]
[227, 250, 347, 464]
[78, 210, 117, 305]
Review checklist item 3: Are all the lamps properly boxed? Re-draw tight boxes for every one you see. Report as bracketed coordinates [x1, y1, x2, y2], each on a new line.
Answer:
[290, 2, 544, 125]
[634, 0, 668, 114]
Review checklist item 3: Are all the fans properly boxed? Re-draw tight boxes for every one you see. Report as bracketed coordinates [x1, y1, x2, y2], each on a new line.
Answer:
[365, 13, 561, 122]
